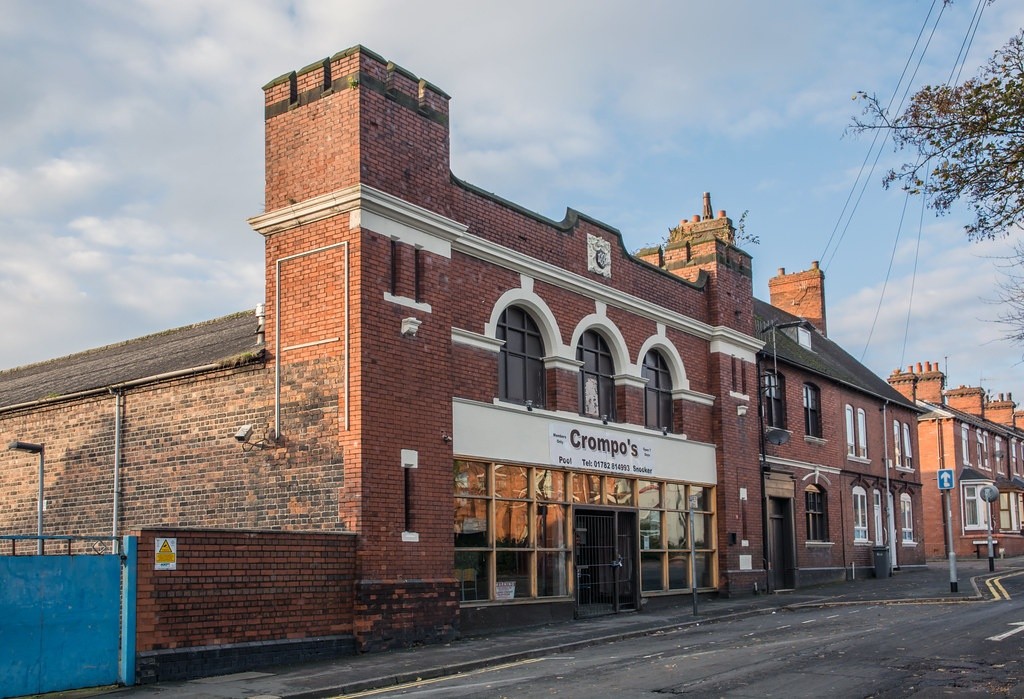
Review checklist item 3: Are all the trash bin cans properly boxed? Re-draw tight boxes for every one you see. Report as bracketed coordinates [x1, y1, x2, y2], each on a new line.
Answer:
[872, 545, 889, 579]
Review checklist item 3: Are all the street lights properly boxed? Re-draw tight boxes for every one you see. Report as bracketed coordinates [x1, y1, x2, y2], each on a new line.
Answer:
[9, 441, 45, 557]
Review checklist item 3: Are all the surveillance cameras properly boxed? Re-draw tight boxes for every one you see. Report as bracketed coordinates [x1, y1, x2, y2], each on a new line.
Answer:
[234, 425, 252, 443]
